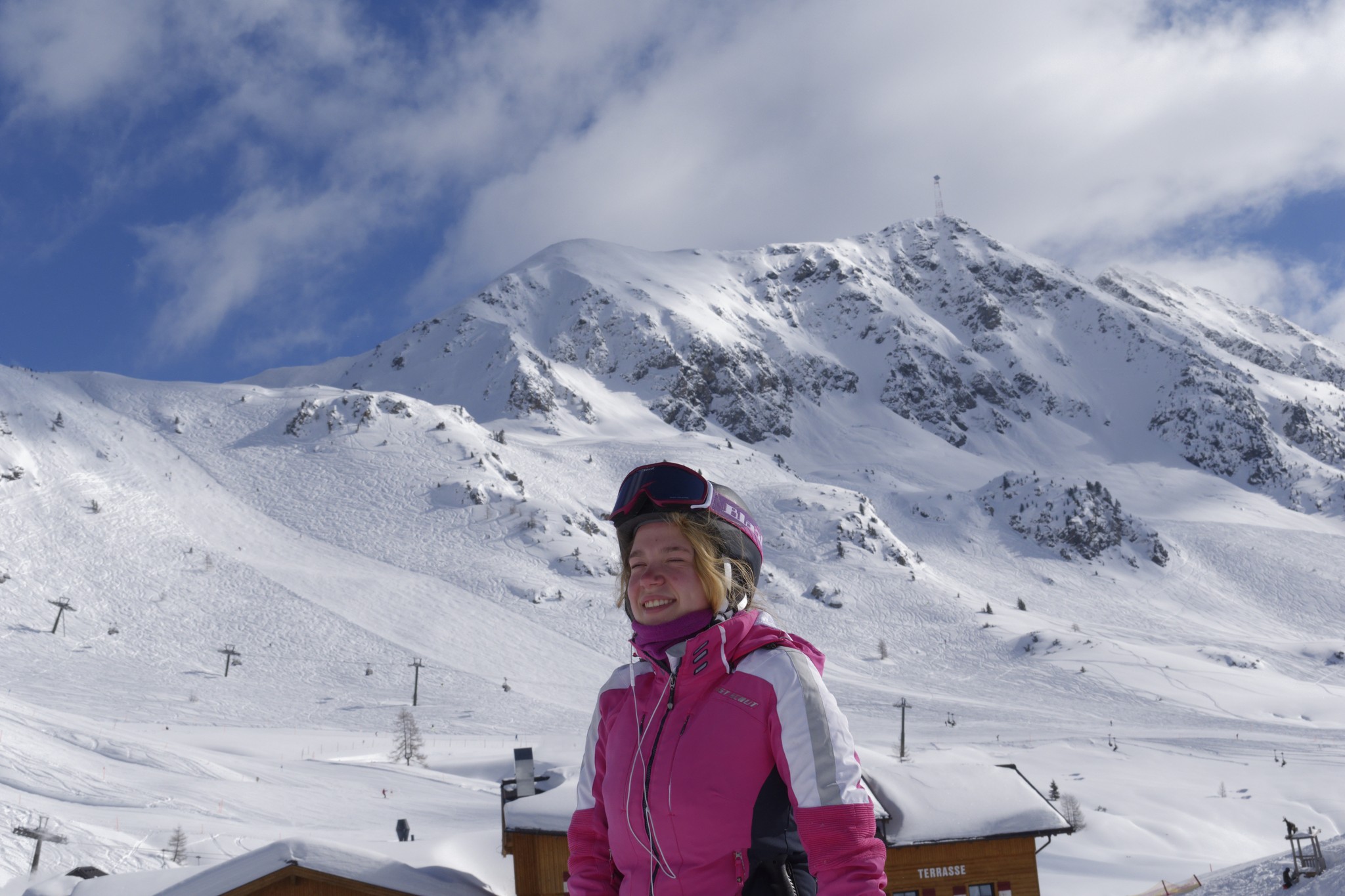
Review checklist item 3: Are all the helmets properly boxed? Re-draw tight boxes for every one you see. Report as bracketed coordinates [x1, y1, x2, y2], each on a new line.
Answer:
[617, 479, 764, 611]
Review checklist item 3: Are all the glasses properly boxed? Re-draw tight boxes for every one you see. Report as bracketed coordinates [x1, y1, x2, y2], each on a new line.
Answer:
[608, 462, 715, 527]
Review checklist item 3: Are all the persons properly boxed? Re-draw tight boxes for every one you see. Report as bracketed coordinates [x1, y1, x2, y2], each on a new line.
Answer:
[563, 461, 889, 896]
